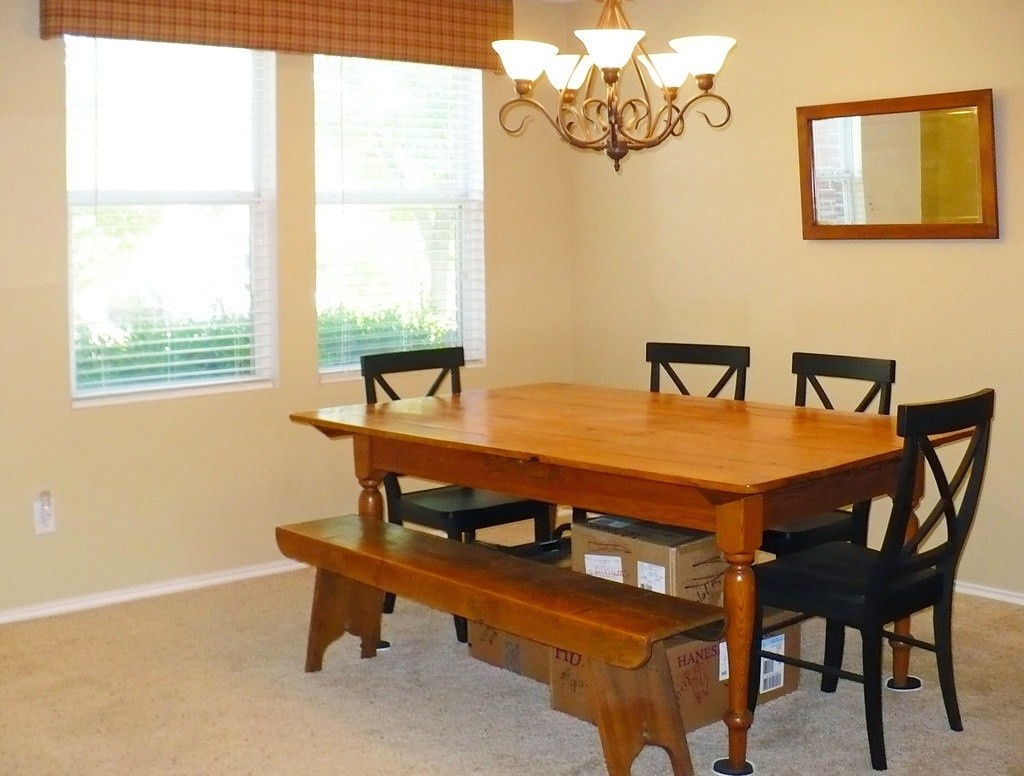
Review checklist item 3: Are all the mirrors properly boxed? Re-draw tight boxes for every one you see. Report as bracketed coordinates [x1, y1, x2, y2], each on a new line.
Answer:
[796, 89, 999, 241]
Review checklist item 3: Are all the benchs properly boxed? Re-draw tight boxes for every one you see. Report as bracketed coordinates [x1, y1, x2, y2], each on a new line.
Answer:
[275, 513, 729, 776]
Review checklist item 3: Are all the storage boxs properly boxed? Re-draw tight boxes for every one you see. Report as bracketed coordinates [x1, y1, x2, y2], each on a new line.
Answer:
[468, 513, 803, 735]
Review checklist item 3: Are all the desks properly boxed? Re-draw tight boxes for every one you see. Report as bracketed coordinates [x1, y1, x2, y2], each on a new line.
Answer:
[291, 381, 976, 775]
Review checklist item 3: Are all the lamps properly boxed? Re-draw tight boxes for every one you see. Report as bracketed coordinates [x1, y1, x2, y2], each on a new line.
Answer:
[492, 0, 737, 172]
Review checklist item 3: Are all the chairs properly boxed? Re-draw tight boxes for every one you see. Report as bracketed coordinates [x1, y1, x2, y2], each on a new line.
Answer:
[752, 388, 995, 771]
[360, 346, 559, 643]
[760, 351, 897, 560]
[644, 341, 751, 401]
